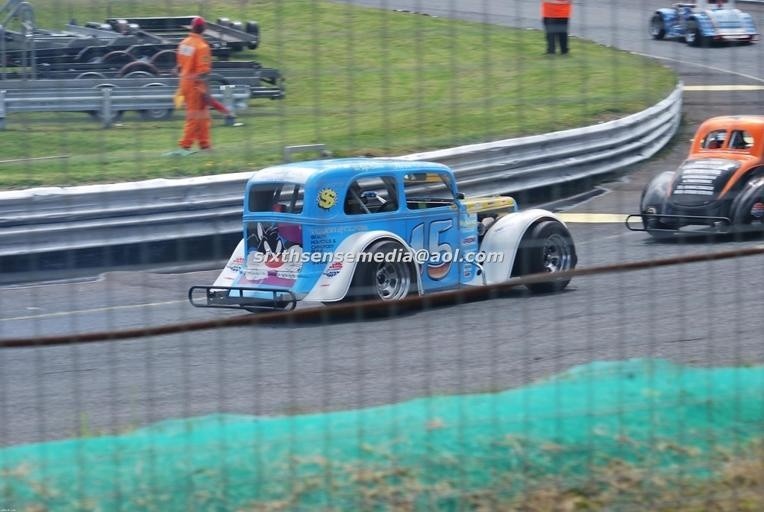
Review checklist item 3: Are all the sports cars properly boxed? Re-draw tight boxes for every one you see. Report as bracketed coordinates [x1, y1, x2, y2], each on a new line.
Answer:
[649, 1, 759, 51]
[184, 150, 582, 323]
[620, 111, 763, 241]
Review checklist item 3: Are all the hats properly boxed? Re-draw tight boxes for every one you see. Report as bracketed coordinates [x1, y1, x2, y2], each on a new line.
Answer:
[192, 17, 204, 29]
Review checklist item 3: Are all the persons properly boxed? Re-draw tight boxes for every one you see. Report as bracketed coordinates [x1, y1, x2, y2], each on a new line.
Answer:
[174, 16, 214, 156]
[540, 0, 571, 56]
